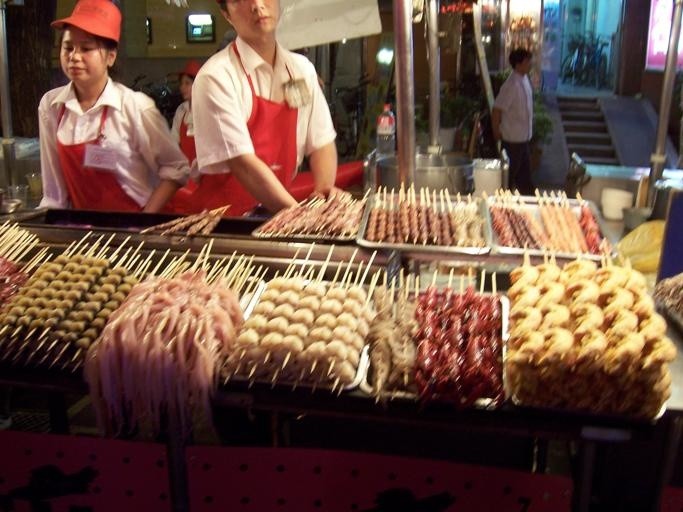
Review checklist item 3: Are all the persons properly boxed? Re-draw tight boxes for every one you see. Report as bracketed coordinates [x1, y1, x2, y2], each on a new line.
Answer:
[189, 1, 340, 216]
[170, 61, 204, 182]
[490, 47, 535, 195]
[32, 0, 193, 216]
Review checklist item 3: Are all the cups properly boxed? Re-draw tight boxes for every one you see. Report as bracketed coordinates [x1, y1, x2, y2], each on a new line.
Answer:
[25, 171, 43, 199]
[9, 184, 29, 211]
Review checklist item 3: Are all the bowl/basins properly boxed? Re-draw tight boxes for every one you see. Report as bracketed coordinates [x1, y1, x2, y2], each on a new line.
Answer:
[600, 186, 633, 221]
[0, 199, 23, 214]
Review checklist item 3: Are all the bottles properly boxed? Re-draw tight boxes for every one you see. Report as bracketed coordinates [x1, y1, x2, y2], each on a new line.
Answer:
[375, 103, 396, 160]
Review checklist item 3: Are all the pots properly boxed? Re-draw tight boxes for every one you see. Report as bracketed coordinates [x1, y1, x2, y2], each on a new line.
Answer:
[377, 152, 476, 197]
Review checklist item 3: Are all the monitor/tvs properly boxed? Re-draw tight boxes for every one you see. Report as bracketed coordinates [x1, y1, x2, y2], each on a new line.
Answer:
[192, 27, 201, 35]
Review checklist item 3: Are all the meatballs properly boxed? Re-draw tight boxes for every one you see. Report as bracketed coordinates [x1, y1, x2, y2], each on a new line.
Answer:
[226, 273, 373, 386]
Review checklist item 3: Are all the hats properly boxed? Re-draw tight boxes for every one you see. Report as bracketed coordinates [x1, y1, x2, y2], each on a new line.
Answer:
[49, 0, 123, 45]
[173, 60, 203, 78]
